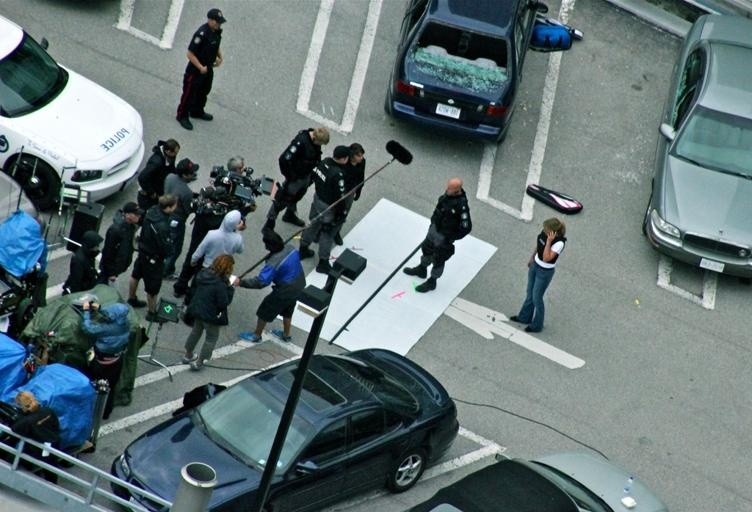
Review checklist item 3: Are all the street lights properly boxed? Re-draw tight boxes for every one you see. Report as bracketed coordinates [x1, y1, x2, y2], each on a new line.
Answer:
[248, 248, 369, 512]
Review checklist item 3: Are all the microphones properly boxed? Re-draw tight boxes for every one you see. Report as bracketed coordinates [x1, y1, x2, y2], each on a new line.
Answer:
[386, 140, 413, 165]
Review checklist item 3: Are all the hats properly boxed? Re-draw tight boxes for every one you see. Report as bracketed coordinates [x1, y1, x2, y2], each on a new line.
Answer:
[207, 9, 227, 23]
[123, 202, 145, 215]
[177, 158, 198, 174]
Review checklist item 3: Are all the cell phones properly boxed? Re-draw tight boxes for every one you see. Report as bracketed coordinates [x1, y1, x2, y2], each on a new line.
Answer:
[554, 230, 558, 236]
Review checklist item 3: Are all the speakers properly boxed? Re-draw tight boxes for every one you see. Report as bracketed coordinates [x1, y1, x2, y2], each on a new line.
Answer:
[66, 203, 104, 252]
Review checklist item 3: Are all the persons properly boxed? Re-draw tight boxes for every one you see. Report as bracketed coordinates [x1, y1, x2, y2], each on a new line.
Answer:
[175, 8, 227, 130]
[511, 218, 566, 332]
[403, 178, 472, 293]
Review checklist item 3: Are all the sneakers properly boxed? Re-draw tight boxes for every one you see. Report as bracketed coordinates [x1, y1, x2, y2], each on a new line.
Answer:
[145, 310, 168, 323]
[403, 264, 427, 278]
[176, 115, 193, 130]
[526, 326, 541, 333]
[271, 329, 291, 343]
[239, 332, 262, 343]
[190, 110, 213, 121]
[261, 219, 275, 235]
[510, 316, 531, 324]
[415, 277, 436, 293]
[182, 352, 208, 370]
[316, 259, 333, 274]
[333, 231, 343, 246]
[298, 245, 314, 260]
[282, 209, 305, 227]
[127, 296, 146, 308]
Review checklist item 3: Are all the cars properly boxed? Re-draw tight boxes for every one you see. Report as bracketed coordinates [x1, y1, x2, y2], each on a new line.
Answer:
[384, 0, 550, 145]
[404, 442, 669, 512]
[643, 9, 752, 278]
[0, 170, 44, 233]
[0, 13, 144, 212]
[109, 347, 464, 512]
